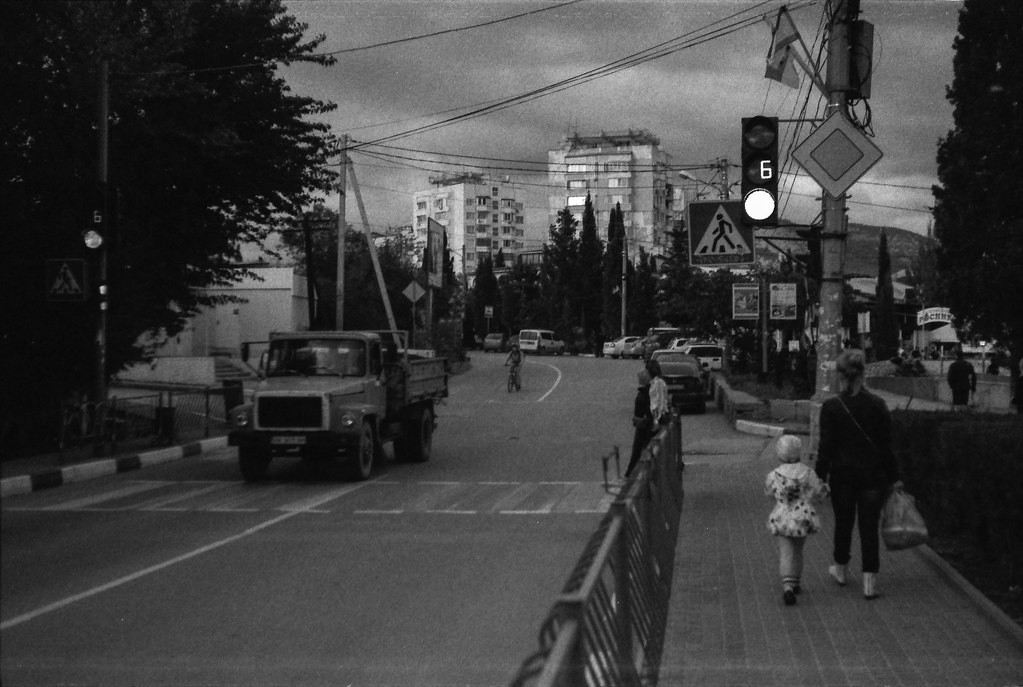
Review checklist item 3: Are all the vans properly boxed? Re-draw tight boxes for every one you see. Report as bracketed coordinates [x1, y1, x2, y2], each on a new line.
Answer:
[520, 330, 565, 355]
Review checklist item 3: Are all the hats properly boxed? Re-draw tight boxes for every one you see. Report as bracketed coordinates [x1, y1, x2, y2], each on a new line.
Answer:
[646, 358, 662, 379]
[512, 343, 518, 347]
[775, 434, 802, 462]
[637, 368, 651, 385]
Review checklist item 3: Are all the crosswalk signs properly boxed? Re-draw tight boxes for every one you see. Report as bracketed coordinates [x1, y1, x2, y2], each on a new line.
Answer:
[688, 202, 756, 266]
[46, 260, 87, 304]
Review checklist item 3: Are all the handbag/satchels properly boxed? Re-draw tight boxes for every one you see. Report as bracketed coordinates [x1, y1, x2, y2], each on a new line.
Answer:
[632, 416, 648, 429]
[968, 391, 980, 407]
[880, 490, 930, 550]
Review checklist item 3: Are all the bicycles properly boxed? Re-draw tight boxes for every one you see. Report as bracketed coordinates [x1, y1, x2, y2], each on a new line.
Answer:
[505, 362, 521, 393]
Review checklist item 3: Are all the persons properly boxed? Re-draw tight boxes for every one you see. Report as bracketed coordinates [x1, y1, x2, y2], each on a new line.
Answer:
[815, 348, 903, 599]
[506, 342, 522, 389]
[912, 346, 922, 370]
[625, 360, 671, 477]
[947, 349, 976, 406]
[764, 435, 831, 605]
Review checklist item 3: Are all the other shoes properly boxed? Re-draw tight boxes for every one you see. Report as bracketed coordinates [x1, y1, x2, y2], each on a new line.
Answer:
[518, 385, 520, 390]
[783, 591, 796, 604]
[793, 586, 800, 593]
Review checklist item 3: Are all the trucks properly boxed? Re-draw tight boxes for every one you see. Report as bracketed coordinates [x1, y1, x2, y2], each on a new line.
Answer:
[225, 329, 451, 481]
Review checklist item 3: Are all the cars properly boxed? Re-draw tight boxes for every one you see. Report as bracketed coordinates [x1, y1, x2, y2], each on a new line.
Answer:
[482, 333, 507, 353]
[504, 335, 519, 352]
[625, 328, 723, 416]
[602, 336, 641, 359]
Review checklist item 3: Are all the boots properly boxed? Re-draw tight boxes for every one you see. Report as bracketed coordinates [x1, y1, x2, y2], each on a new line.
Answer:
[863, 572, 881, 599]
[829, 562, 848, 585]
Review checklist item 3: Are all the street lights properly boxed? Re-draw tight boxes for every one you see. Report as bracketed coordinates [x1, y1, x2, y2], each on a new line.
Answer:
[600, 238, 626, 336]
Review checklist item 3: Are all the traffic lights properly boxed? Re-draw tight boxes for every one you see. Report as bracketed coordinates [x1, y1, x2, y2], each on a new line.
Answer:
[82, 186, 108, 252]
[740, 116, 779, 227]
